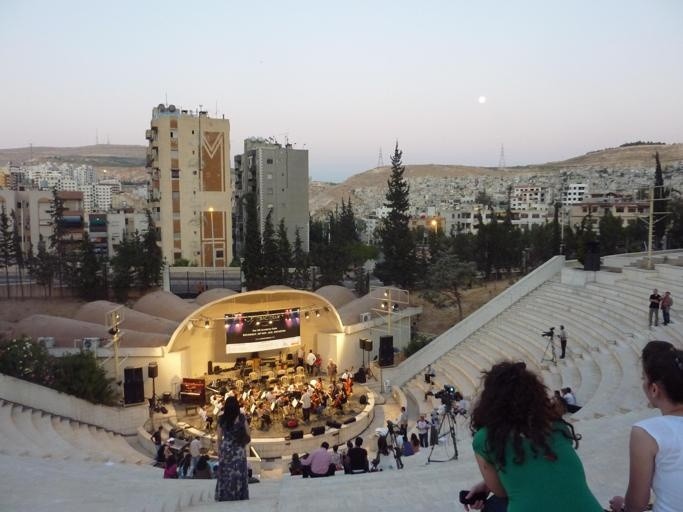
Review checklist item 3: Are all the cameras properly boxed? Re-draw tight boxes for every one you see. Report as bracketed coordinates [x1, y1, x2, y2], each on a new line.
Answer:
[386, 420, 393, 427]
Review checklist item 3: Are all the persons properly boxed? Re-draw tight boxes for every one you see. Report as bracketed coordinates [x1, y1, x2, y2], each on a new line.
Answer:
[462, 356, 606, 511]
[150, 346, 467, 479]
[410, 321, 419, 340]
[550, 387, 576, 416]
[609, 340, 683, 512]
[658, 291, 673, 324]
[556, 325, 568, 358]
[648, 288, 661, 327]
[212, 395, 251, 502]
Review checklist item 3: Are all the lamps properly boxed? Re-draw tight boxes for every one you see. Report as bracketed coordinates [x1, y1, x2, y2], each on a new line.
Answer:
[204, 307, 329, 329]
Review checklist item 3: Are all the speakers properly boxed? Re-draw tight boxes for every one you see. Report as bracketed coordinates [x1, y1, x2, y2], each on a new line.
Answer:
[364, 340, 373, 351]
[148, 365, 158, 378]
[124, 382, 145, 405]
[380, 335, 393, 348]
[123, 367, 143, 382]
[378, 348, 394, 366]
[360, 339, 368, 348]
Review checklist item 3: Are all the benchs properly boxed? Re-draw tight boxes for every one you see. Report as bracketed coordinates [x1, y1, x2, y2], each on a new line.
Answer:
[0, 399, 216, 511]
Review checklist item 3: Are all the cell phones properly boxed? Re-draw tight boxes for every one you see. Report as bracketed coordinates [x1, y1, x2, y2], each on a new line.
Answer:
[459, 490, 488, 506]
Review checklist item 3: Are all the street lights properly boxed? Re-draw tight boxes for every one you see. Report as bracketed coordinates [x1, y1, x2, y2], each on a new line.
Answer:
[208, 208, 218, 271]
[429, 218, 439, 235]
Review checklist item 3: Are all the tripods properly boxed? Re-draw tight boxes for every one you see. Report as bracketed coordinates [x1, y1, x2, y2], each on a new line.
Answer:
[427, 404, 459, 463]
[541, 336, 557, 367]
[370, 428, 404, 472]
[149, 378, 162, 410]
[363, 351, 377, 381]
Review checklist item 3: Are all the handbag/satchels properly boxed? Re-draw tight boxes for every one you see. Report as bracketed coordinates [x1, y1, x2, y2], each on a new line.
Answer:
[233, 423, 250, 446]
